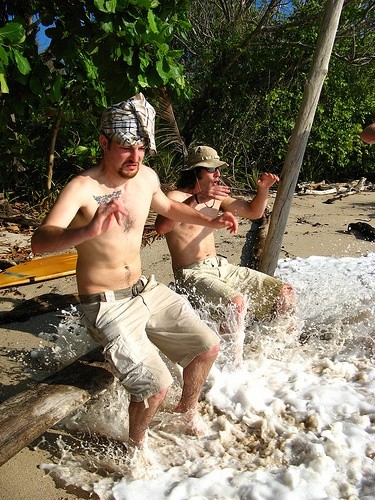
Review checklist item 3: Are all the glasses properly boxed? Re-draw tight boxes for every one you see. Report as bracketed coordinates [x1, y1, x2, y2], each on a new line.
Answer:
[203, 167, 224, 173]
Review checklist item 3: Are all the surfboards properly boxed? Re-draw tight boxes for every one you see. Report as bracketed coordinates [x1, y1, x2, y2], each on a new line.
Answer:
[0, 250, 77, 288]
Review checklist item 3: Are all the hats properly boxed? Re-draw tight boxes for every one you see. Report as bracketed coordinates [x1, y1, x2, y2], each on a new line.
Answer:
[99, 93, 158, 151]
[182, 146, 229, 171]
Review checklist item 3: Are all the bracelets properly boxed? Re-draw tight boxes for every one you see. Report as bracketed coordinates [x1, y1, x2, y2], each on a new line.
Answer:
[195, 194, 199, 204]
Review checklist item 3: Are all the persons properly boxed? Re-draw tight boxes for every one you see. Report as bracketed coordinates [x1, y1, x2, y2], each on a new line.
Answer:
[154, 145, 295, 335]
[30, 92, 238, 451]
[360, 123, 375, 144]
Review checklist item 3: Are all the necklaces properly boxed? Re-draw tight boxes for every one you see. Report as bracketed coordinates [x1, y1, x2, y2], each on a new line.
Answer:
[204, 199, 215, 208]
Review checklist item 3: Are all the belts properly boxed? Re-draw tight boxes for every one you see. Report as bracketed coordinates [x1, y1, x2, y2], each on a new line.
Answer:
[78, 271, 150, 305]
